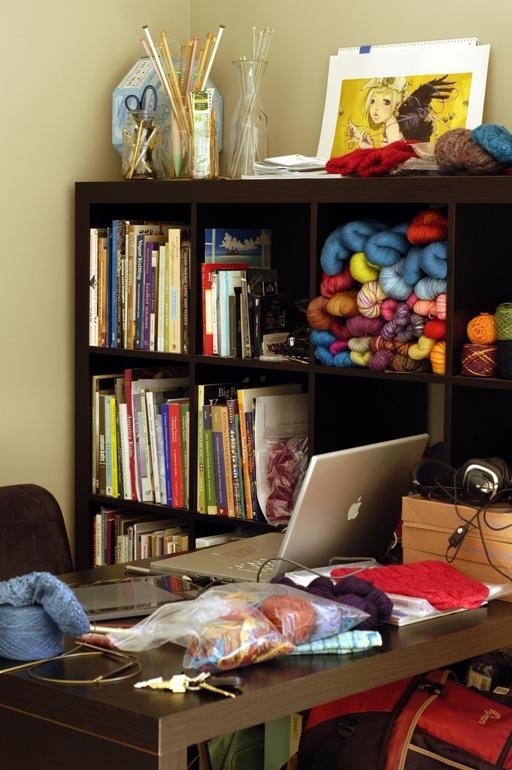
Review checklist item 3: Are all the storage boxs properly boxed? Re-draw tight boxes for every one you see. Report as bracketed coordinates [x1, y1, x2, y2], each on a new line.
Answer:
[401, 493, 512, 605]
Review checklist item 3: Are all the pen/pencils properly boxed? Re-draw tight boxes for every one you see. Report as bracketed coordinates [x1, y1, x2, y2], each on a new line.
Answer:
[124, 564, 193, 582]
[123, 119, 158, 181]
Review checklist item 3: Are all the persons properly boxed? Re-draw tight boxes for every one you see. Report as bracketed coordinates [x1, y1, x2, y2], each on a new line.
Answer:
[345, 76, 408, 150]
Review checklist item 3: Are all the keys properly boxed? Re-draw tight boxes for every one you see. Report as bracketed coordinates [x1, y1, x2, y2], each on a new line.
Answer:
[206, 675, 243, 690]
[133, 674, 190, 693]
[201, 682, 236, 699]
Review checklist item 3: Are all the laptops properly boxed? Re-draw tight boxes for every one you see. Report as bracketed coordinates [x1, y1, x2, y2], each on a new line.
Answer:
[149, 433, 431, 582]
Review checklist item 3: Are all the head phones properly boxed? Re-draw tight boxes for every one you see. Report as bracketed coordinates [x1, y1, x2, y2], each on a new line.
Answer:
[410, 455, 512, 506]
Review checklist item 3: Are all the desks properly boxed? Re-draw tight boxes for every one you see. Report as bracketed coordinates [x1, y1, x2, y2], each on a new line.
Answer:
[0, 540, 512, 770]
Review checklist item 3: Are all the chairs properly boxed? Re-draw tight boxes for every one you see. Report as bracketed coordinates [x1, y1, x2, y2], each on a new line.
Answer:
[0, 483, 74, 585]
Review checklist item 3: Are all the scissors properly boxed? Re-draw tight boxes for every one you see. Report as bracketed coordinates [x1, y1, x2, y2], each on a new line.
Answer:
[124, 85, 157, 170]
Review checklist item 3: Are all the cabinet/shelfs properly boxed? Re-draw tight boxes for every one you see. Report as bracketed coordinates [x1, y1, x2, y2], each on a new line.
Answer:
[77, 176, 512, 577]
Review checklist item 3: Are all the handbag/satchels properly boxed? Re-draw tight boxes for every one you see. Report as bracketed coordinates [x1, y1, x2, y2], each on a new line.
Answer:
[296, 669, 512, 770]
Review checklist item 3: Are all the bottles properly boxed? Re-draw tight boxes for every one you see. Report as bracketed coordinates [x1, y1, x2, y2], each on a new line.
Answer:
[121, 94, 193, 180]
[227, 61, 270, 179]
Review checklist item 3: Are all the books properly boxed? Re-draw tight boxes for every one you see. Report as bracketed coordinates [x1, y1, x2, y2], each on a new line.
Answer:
[393, 157, 443, 175]
[90, 220, 288, 358]
[282, 559, 505, 627]
[240, 152, 351, 181]
[92, 368, 309, 523]
[94, 509, 242, 567]
[336, 36, 481, 57]
